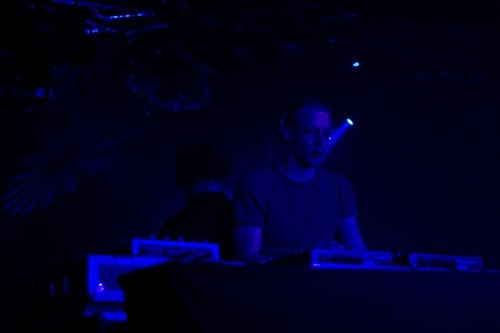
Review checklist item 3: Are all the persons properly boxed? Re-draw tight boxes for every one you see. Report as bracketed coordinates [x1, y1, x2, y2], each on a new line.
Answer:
[235, 97, 367, 267]
[156, 143, 239, 272]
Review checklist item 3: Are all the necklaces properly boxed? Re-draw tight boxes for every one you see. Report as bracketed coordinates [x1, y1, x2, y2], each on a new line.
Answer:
[282, 166, 322, 249]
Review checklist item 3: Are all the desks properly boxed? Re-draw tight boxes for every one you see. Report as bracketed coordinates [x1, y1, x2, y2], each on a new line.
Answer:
[118, 264, 500, 333]
